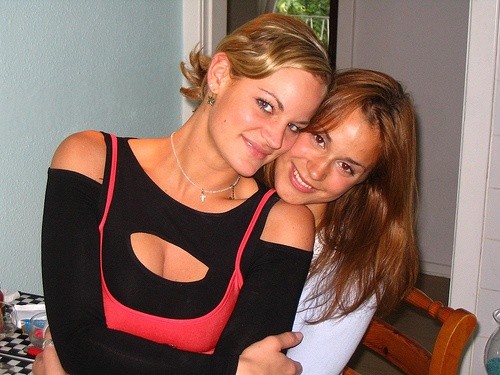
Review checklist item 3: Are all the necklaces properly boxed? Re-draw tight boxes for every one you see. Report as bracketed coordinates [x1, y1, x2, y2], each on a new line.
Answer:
[171, 131, 241, 202]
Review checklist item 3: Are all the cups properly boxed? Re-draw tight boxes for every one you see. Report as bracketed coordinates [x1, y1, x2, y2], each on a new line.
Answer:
[28, 312, 49, 348]
[0, 301, 17, 339]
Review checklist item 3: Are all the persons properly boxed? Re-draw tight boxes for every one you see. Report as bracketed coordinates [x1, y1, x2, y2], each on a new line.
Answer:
[42, 67, 420, 375]
[32, 13, 332, 375]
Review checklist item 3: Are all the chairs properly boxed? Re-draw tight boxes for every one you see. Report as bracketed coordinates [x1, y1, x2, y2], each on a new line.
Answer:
[338, 287, 477, 375]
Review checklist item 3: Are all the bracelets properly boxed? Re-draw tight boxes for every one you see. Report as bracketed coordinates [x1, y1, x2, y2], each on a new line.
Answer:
[41, 338, 55, 351]
